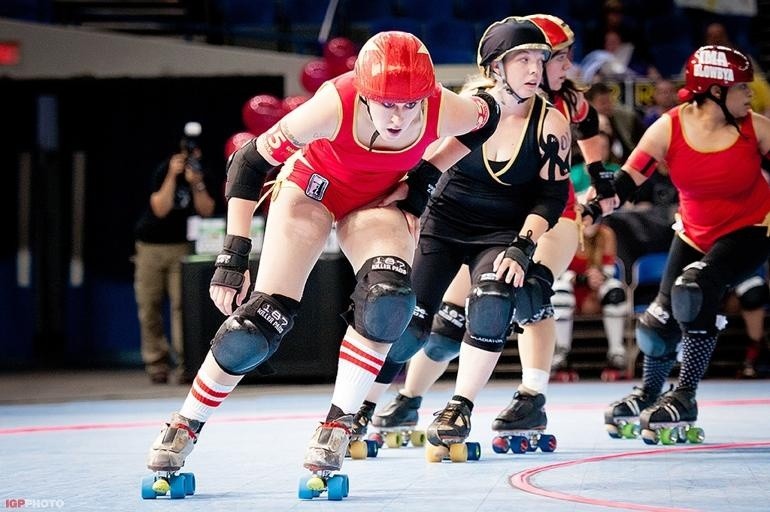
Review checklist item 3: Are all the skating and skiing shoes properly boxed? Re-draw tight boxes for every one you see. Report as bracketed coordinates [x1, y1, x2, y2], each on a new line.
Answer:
[345, 400, 378, 460]
[298, 404, 356, 501]
[426, 400, 480, 462]
[491, 383, 556, 453]
[640, 384, 704, 445]
[605, 386, 664, 438]
[141, 412, 206, 500]
[549, 346, 634, 383]
[367, 388, 425, 449]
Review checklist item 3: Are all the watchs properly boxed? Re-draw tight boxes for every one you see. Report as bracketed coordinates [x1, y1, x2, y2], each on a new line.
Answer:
[191, 181, 205, 193]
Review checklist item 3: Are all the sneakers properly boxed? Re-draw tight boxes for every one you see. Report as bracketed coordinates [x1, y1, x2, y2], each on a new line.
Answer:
[151, 371, 167, 384]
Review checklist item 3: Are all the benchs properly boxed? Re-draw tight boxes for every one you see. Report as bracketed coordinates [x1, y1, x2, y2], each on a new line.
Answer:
[447, 313, 769, 375]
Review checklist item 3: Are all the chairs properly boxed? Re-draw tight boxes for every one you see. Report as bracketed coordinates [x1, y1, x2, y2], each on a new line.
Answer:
[630, 252, 670, 379]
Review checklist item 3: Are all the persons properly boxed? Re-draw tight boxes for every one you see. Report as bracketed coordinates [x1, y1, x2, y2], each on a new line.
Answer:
[534, 1, 684, 370]
[129, 137, 215, 383]
[365, 15, 616, 452]
[703, 21, 770, 116]
[340, 18, 572, 458]
[723, 266, 770, 379]
[140, 28, 501, 499]
[578, 47, 770, 444]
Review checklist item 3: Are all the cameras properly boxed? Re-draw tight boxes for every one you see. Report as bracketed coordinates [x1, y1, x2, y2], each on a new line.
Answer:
[182, 158, 203, 173]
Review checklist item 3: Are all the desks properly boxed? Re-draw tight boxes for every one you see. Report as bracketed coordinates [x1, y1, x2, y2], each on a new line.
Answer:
[179, 255, 353, 383]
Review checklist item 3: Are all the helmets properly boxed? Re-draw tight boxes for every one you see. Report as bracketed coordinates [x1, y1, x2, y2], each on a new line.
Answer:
[523, 14, 576, 52]
[683, 45, 753, 102]
[477, 16, 553, 80]
[351, 30, 436, 104]
